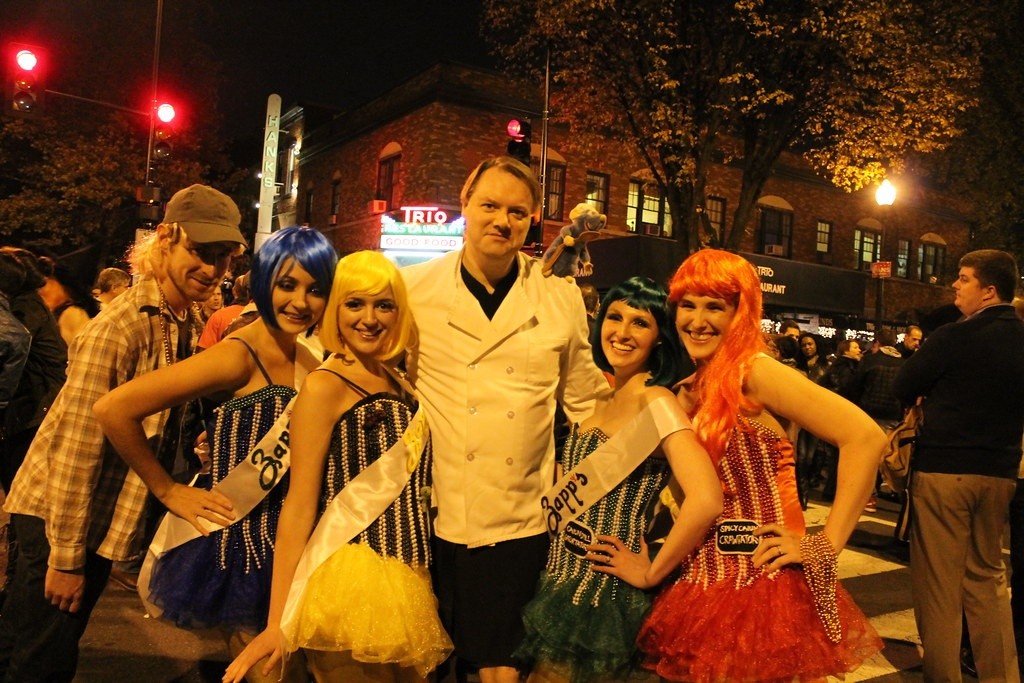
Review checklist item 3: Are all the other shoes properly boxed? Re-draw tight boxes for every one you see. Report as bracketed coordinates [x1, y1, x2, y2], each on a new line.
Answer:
[960, 649, 977, 676]
[864, 497, 878, 514]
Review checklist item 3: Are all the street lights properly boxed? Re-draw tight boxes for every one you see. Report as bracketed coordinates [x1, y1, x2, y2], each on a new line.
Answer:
[875, 179, 896, 332]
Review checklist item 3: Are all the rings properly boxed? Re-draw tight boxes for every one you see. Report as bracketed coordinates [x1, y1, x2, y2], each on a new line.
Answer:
[778, 546, 783, 554]
[605, 557, 610, 563]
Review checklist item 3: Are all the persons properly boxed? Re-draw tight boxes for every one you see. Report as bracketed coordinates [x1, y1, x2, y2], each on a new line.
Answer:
[509, 275, 724, 683]
[775, 320, 923, 505]
[399, 154, 612, 683]
[667, 249, 900, 683]
[775, 248, 1024, 683]
[0, 182, 250, 683]
[91, 226, 338, 683]
[221, 249, 457, 683]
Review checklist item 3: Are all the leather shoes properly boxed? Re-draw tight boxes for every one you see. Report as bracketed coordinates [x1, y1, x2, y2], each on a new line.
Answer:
[109, 562, 139, 592]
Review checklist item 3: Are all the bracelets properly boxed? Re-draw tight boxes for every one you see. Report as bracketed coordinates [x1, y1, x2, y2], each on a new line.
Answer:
[800, 531, 841, 645]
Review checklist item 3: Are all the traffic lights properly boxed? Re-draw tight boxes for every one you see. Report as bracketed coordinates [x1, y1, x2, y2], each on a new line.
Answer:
[149, 103, 178, 164]
[506, 118, 531, 168]
[10, 49, 39, 114]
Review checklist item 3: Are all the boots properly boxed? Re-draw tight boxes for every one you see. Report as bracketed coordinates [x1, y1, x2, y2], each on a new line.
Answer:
[797, 459, 808, 508]
[810, 450, 825, 488]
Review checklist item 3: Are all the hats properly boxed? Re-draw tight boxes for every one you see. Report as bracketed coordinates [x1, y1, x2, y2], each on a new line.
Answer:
[163, 184, 248, 251]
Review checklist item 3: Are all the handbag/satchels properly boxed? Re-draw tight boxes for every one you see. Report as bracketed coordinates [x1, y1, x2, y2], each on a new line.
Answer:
[874, 404, 924, 492]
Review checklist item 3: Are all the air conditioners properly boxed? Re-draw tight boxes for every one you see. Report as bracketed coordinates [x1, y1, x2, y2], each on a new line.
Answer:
[644, 224, 660, 236]
[367, 200, 386, 216]
[329, 215, 337, 224]
[765, 245, 784, 256]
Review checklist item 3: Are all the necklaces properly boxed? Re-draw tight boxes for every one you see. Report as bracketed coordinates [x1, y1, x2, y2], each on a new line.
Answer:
[159, 287, 200, 366]
